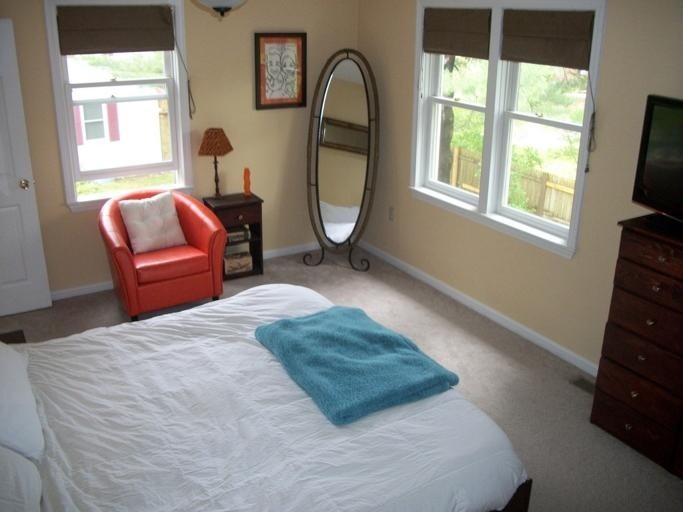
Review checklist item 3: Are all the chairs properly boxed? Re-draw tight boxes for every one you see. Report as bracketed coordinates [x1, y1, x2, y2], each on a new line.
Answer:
[98, 185, 229, 321]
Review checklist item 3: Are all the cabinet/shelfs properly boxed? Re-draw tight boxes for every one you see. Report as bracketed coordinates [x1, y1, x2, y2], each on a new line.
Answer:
[589, 211, 683, 484]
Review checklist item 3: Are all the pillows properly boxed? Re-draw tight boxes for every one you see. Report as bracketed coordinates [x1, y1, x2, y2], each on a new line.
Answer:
[0, 337, 46, 509]
[116, 191, 190, 255]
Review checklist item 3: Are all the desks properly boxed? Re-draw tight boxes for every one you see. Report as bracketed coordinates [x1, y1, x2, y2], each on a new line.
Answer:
[203, 191, 266, 282]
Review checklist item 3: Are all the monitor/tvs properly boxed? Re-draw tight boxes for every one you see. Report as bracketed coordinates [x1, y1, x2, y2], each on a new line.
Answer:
[633, 95, 683, 235]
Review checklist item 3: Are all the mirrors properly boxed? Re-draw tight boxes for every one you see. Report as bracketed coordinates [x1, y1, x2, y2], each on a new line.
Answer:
[300, 47, 378, 274]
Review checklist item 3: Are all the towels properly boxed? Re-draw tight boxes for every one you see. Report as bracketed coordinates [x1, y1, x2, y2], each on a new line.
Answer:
[253, 302, 460, 426]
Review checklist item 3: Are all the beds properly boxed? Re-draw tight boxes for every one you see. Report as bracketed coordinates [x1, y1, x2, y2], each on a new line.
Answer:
[0, 283, 533, 511]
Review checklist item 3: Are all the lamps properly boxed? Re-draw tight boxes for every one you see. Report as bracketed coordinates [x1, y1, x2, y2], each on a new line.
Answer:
[195, 126, 234, 199]
[196, 0, 246, 18]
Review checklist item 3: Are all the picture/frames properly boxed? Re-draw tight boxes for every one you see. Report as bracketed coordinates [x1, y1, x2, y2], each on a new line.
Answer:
[252, 31, 309, 111]
